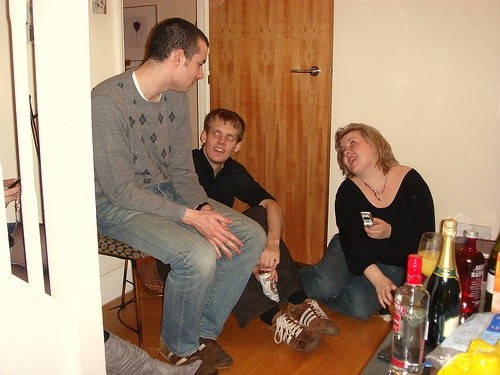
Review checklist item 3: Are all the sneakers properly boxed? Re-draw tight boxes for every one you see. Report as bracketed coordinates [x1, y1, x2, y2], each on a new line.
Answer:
[285, 297, 340, 336]
[158, 336, 219, 375]
[266, 309, 323, 352]
[194, 337, 234, 369]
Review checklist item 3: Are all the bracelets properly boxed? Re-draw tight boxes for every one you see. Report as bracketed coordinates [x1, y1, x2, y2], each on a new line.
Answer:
[197, 202, 209, 211]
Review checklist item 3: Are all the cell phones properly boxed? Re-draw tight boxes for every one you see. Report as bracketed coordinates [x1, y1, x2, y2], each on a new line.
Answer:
[360, 211, 376, 228]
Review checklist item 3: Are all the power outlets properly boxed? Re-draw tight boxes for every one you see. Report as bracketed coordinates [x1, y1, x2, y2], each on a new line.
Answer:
[474, 225, 491, 242]
[457, 223, 473, 240]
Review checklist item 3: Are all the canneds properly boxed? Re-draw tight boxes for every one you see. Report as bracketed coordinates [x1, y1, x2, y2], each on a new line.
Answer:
[258, 271, 280, 303]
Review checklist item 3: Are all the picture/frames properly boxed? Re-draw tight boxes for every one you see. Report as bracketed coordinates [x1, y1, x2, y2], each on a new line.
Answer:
[91, 0, 106, 15]
[123, 4, 158, 62]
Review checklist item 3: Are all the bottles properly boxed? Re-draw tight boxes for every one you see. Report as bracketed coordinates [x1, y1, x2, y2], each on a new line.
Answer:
[454, 229, 486, 320]
[387, 253, 431, 374]
[484, 235, 500, 312]
[424, 219, 462, 346]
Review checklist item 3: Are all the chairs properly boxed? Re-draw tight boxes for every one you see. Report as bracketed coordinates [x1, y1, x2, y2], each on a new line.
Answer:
[99, 234, 166, 349]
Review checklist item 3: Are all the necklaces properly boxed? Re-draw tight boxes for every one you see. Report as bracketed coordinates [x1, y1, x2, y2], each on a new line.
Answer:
[364, 175, 387, 201]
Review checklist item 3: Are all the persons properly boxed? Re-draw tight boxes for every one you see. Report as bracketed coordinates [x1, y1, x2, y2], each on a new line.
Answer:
[292, 123, 435, 321]
[3, 177, 202, 375]
[90, 18, 267, 375]
[156, 108, 340, 352]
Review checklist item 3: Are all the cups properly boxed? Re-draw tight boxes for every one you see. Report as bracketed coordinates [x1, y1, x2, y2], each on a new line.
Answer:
[417, 232, 443, 277]
